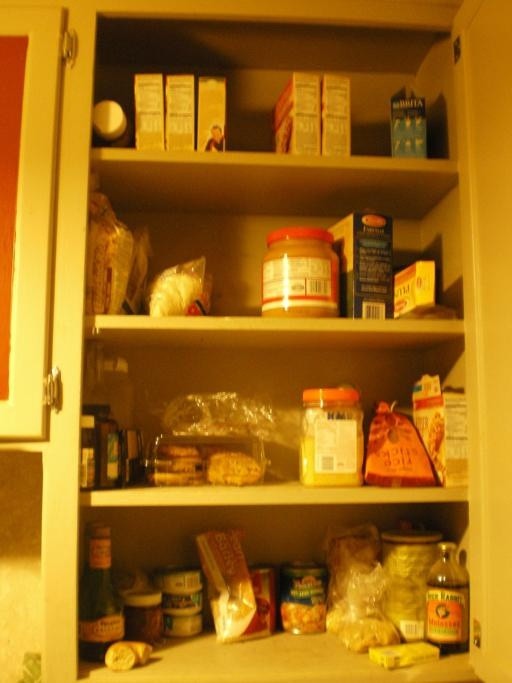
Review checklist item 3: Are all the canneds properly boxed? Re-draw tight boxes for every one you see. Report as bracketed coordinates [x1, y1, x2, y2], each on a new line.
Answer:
[281, 560, 328, 635]
[122, 566, 206, 645]
[246, 562, 277, 633]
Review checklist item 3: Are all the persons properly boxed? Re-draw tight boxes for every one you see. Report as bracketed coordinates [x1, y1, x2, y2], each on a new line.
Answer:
[203, 125, 223, 152]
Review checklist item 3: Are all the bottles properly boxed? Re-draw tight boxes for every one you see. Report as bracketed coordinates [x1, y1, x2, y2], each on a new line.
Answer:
[298, 387, 365, 488]
[423, 540, 470, 655]
[120, 587, 164, 652]
[79, 403, 145, 491]
[78, 521, 127, 663]
[92, 99, 134, 148]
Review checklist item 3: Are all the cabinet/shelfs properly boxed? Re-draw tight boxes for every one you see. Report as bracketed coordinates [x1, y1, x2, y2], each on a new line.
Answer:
[1, 0, 76, 455]
[42, 0, 512, 682]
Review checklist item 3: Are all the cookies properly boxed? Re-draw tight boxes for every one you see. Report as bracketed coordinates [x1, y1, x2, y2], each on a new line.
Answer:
[150, 446, 262, 485]
